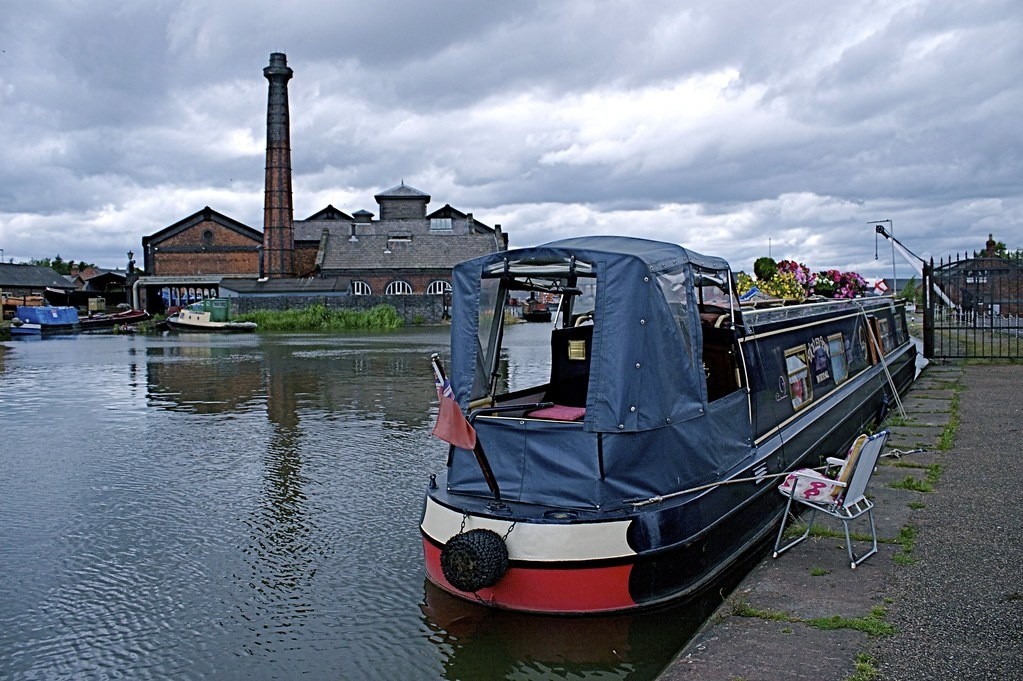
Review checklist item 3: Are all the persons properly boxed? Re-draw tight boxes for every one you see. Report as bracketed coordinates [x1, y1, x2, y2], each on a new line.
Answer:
[527, 292, 538, 304]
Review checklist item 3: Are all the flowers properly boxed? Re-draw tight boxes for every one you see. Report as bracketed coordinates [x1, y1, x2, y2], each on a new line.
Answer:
[735, 260, 869, 304]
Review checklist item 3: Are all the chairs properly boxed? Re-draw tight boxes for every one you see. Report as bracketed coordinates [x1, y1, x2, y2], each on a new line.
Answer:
[773, 429, 891, 570]
[524, 324, 593, 422]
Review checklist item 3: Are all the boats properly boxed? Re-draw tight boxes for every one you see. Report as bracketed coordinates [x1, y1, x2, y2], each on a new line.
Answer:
[418, 236, 918, 612]
[166, 294, 260, 333]
[76, 309, 150, 326]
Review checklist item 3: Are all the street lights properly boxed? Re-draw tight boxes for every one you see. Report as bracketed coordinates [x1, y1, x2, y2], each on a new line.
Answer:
[127, 251, 134, 275]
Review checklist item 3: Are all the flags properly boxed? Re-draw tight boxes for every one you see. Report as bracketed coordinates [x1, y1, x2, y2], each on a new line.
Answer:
[433, 378, 475, 449]
